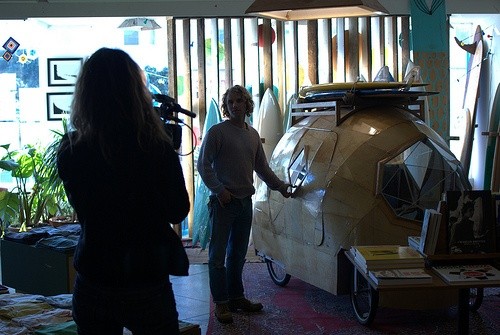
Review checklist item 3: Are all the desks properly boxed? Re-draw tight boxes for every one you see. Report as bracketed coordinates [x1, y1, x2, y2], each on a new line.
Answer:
[345, 250, 500, 326]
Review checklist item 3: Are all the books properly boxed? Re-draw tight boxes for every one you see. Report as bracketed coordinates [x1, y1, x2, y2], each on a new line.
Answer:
[349, 245, 500, 286]
[408, 208, 441, 255]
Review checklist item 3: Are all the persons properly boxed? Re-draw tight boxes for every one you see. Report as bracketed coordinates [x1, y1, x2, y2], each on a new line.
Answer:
[197, 85, 294, 322]
[56, 47, 190, 334]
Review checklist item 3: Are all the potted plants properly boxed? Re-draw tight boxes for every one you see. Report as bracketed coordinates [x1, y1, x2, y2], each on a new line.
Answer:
[0, 117, 80, 236]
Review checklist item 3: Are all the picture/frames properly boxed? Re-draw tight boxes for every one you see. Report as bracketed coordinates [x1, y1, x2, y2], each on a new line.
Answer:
[47, 57, 84, 87]
[45, 92, 74, 120]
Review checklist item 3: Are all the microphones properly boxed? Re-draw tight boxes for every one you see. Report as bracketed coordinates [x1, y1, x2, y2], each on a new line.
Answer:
[165, 102, 196, 118]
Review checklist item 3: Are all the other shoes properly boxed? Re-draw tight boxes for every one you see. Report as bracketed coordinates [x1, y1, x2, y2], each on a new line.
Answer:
[214, 301, 234, 324]
[233, 296, 264, 313]
